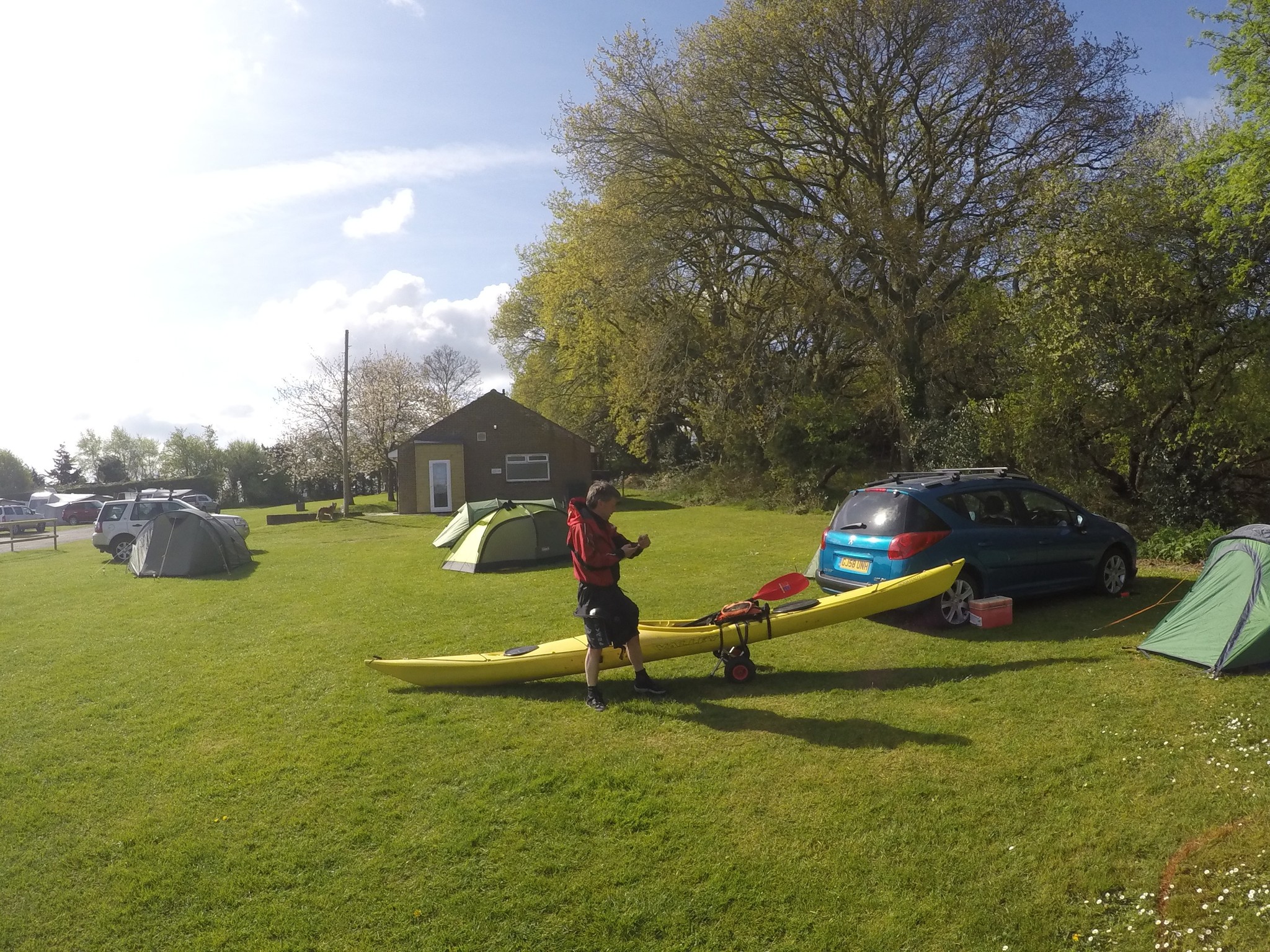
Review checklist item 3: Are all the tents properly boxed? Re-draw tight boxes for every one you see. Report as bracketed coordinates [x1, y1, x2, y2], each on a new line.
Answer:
[432, 498, 573, 574]
[1136, 523, 1270, 679]
[802, 500, 840, 579]
[127, 508, 253, 579]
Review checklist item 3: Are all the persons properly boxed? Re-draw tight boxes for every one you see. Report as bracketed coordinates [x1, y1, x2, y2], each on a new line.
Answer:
[566, 481, 667, 712]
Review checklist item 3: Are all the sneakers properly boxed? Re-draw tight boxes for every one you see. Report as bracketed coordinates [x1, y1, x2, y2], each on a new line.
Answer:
[585, 694, 605, 712]
[634, 678, 666, 694]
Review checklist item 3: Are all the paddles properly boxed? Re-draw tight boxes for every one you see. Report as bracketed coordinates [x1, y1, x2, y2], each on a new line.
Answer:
[678, 572, 809, 627]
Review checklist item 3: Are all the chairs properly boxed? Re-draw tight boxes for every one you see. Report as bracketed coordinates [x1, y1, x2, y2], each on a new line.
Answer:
[980, 496, 1012, 526]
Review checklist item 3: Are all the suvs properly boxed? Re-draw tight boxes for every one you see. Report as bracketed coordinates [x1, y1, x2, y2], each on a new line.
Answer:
[0, 504, 47, 536]
[815, 464, 1139, 628]
[62, 499, 122, 526]
[181, 493, 221, 514]
[91, 492, 250, 561]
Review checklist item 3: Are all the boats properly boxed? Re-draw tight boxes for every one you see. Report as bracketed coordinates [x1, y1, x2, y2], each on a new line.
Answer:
[362, 556, 967, 689]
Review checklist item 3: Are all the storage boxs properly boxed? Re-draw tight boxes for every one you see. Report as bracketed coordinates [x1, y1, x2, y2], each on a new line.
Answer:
[969, 596, 1013, 628]
